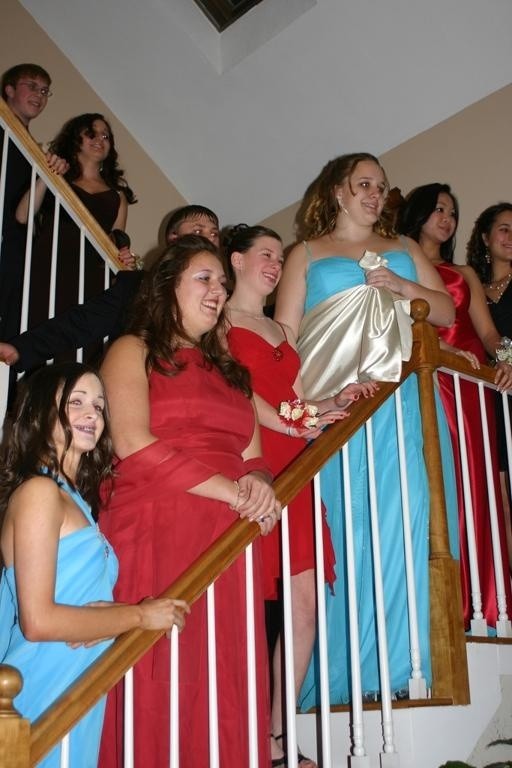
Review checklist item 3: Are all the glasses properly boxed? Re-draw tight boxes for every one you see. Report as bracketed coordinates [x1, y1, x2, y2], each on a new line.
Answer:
[16, 80, 53, 97]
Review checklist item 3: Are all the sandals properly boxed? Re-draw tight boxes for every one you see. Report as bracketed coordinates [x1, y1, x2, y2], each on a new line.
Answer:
[269, 731, 313, 767]
